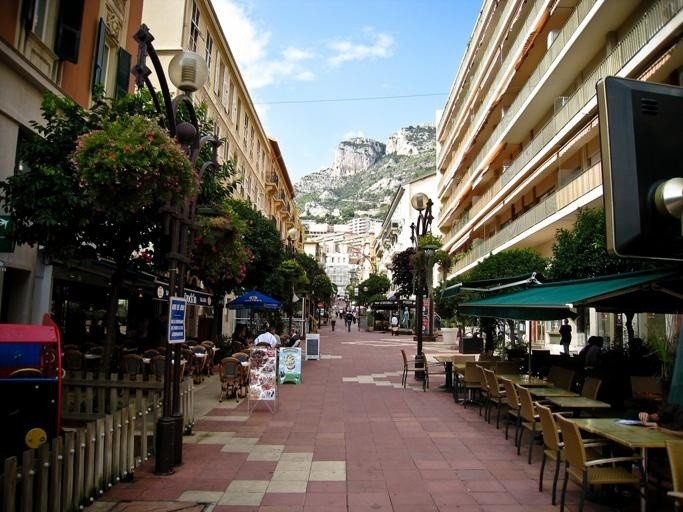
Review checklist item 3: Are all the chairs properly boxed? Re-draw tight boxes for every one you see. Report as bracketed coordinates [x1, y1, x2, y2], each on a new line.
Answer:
[400, 348, 429, 392]
[67, 332, 303, 403]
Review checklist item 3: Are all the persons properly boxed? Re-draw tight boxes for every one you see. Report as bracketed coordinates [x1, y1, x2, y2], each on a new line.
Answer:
[391, 315, 398, 336]
[479, 344, 494, 361]
[329, 307, 357, 332]
[579, 336, 656, 367]
[233, 323, 301, 349]
[639, 404, 683, 437]
[559, 319, 572, 355]
[434, 315, 440, 328]
[402, 307, 409, 329]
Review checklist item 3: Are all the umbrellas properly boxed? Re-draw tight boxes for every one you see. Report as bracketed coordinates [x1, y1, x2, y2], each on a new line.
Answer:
[226, 288, 281, 335]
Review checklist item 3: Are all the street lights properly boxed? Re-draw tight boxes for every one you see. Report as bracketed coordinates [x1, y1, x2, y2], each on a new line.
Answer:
[363, 286, 368, 316]
[402, 192, 438, 379]
[129, 19, 220, 472]
[284, 227, 301, 334]
[350, 248, 372, 274]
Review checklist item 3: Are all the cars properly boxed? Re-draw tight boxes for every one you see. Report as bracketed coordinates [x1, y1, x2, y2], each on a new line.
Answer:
[318, 309, 326, 316]
